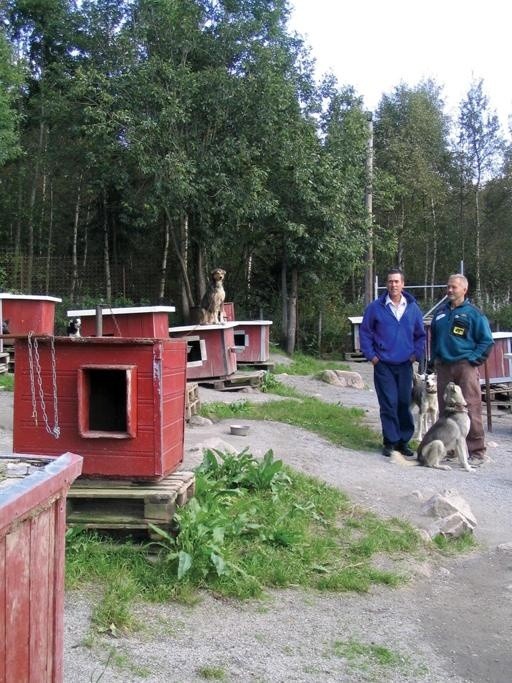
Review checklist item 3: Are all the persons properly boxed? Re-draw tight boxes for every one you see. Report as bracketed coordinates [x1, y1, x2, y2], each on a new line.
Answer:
[359, 268, 427, 457]
[426, 273, 494, 468]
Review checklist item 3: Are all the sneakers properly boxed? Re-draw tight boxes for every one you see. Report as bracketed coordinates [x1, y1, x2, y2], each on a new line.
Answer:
[467, 455, 489, 468]
[381, 446, 413, 457]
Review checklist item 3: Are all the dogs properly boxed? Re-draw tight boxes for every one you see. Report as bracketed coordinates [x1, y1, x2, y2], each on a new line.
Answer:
[409, 372, 439, 442]
[199, 267, 226, 325]
[2, 318, 11, 334]
[67, 318, 82, 337]
[390, 381, 477, 473]
